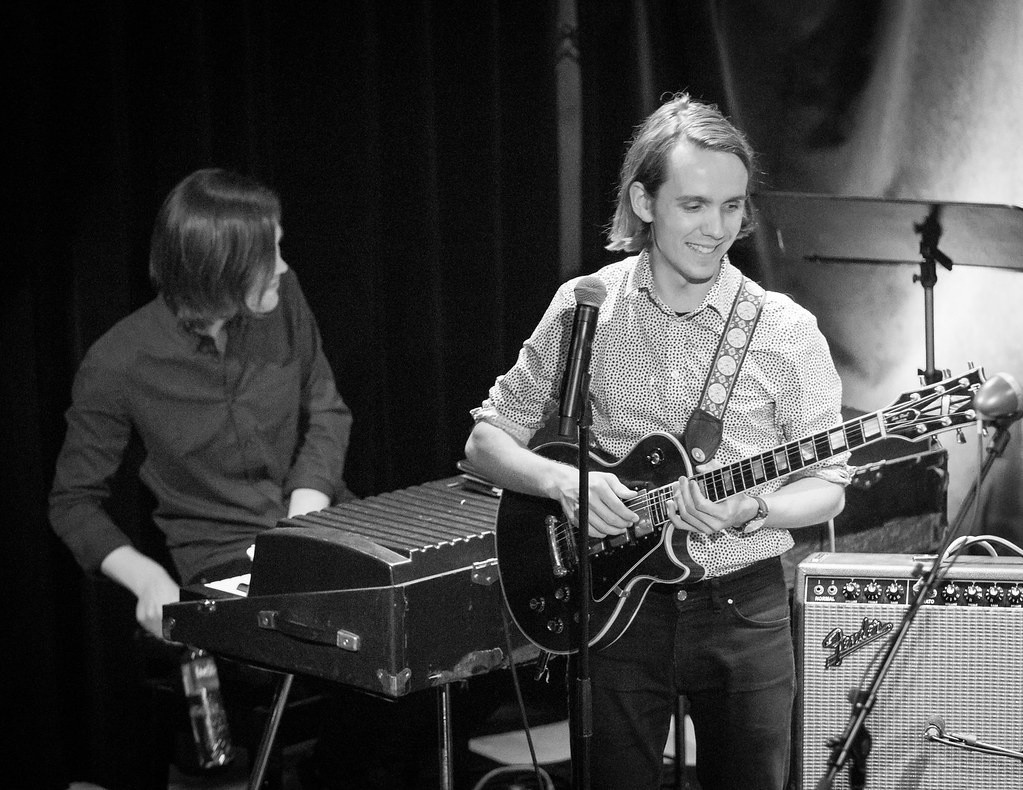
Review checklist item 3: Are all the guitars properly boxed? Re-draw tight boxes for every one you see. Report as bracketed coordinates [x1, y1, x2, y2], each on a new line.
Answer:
[494, 361, 989, 655]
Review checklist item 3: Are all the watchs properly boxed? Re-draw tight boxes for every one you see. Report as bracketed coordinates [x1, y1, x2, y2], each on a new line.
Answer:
[732, 496, 769, 534]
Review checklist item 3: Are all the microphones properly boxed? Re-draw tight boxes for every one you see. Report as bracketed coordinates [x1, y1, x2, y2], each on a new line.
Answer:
[558, 278, 608, 439]
[973, 371, 1023, 423]
[925, 715, 945, 742]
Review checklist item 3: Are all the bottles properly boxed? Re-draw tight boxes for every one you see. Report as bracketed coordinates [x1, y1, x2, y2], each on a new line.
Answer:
[179, 645, 235, 769]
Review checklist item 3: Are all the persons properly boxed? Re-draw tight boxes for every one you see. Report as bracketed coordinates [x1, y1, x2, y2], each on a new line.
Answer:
[463, 94, 852, 790]
[49, 168, 354, 648]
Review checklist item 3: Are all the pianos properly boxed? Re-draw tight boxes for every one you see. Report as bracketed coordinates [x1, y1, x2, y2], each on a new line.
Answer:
[162, 404, 949, 701]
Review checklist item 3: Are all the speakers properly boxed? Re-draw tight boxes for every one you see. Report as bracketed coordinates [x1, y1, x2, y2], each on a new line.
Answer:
[794, 551, 1023, 790]
